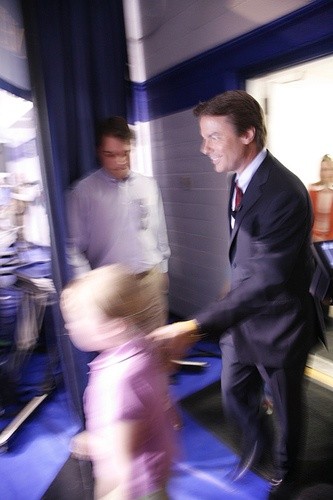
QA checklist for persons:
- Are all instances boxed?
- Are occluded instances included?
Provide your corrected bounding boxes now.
[60,120,169,348]
[58,261,180,500]
[139,88,328,500]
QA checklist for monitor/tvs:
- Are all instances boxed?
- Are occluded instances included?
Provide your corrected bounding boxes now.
[307,238,333,307]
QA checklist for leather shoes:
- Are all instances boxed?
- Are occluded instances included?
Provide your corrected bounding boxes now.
[266,461,302,500]
[228,434,274,487]
